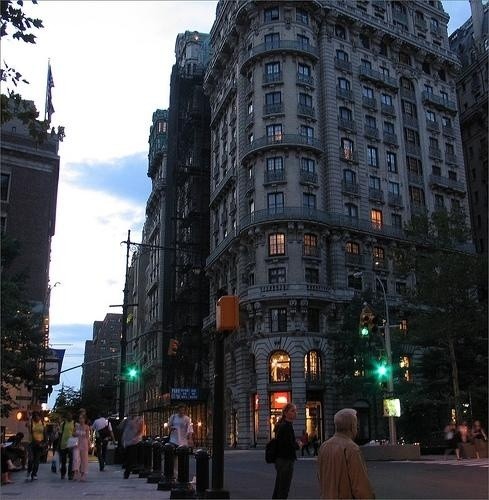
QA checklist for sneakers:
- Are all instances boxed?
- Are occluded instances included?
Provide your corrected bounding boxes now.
[52,463,87,481]
[2,466,38,486]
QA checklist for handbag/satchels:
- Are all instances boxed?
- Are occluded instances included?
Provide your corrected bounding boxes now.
[67,437,79,449]
[98,428,110,440]
[265,439,279,464]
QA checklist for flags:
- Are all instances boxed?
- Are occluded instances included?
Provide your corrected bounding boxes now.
[46,62,55,123]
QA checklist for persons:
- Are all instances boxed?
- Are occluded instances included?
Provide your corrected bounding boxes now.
[299,429,318,457]
[0,408,94,484]
[316,409,374,499]
[167,406,194,480]
[92,410,117,471]
[442,418,487,461]
[272,404,300,499]
[119,410,145,479]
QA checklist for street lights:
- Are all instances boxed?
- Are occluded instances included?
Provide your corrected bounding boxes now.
[353,270,398,445]
